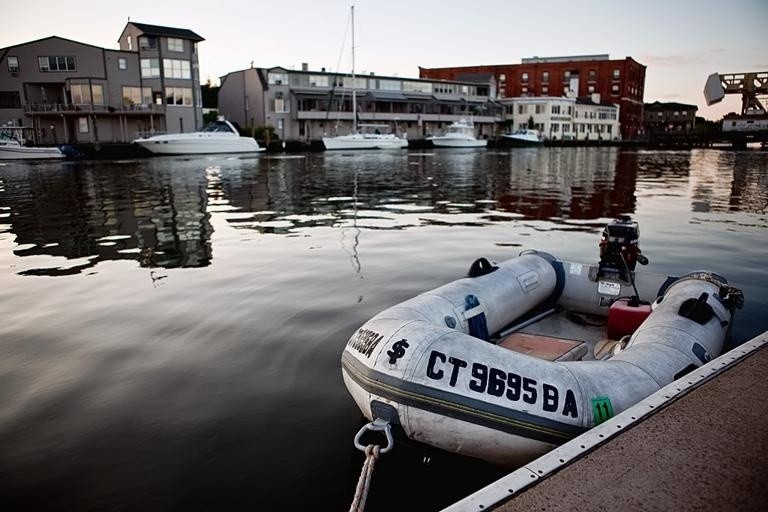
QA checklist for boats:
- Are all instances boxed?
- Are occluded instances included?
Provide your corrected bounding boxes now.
[130,116,267,155]
[425,115,488,148]
[0,121,67,160]
[342,249,745,466]
[496,129,541,146]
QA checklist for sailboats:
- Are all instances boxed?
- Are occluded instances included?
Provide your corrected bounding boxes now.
[321,6,409,150]
